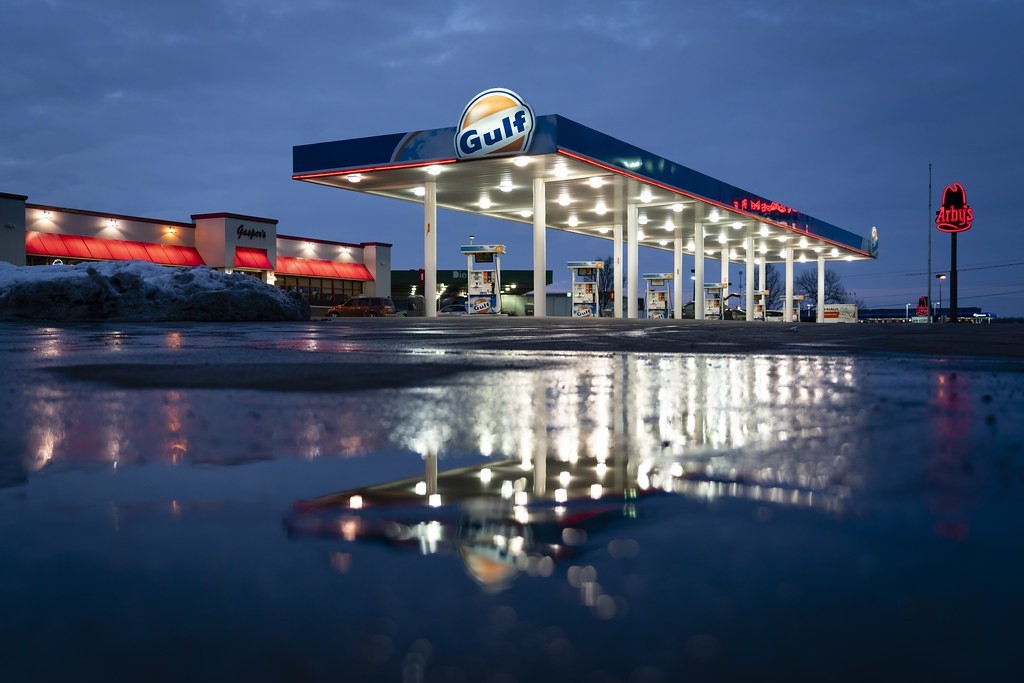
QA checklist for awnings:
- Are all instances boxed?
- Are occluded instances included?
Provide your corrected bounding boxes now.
[274,254,375,282]
[233,247,274,271]
[25,230,207,268]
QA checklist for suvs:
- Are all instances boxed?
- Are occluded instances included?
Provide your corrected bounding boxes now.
[326,297,396,318]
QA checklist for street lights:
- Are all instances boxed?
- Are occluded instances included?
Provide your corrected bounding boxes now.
[933,300,939,322]
[905,301,912,320]
[938,273,946,316]
[689,275,697,303]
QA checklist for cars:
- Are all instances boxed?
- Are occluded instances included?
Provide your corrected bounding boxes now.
[437,301,471,316]
